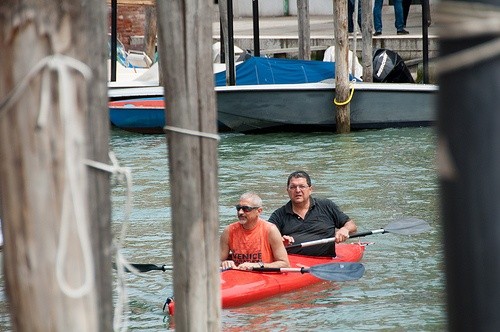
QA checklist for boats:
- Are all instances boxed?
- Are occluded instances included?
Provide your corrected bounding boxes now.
[168,242,375,315]
[108,84,438,134]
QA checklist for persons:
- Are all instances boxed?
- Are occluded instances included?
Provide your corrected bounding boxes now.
[268,171,357,257]
[348,0,362,35]
[218,193,290,271]
[372,0,409,36]
[402,0,431,27]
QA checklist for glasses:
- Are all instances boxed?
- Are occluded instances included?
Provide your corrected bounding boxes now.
[287,184,309,191]
[236,205,259,212]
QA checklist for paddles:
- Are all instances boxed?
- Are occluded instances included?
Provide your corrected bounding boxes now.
[111,261,365,282]
[284,216,430,251]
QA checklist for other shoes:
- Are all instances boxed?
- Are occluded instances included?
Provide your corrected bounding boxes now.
[397,29,410,35]
[372,31,382,36]
